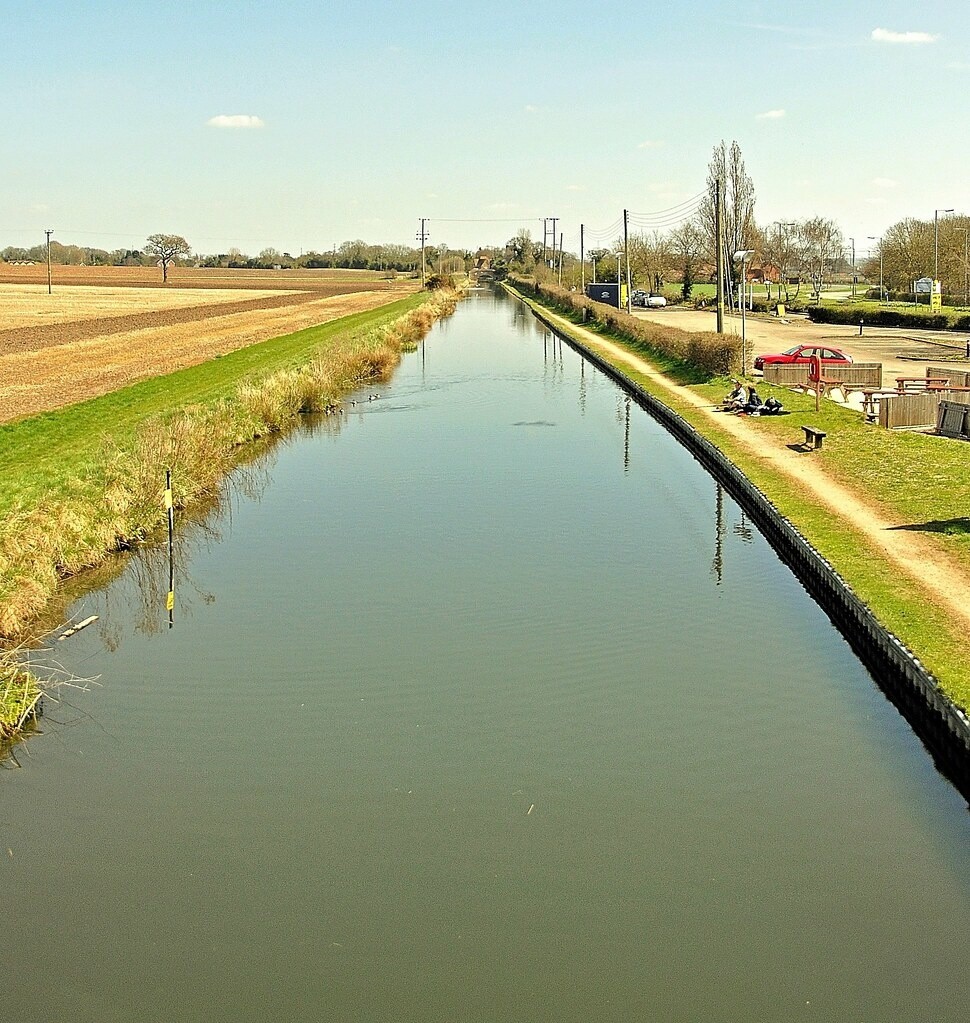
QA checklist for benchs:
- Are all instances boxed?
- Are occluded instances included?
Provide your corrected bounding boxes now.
[867,397,880,401]
[801,426,827,449]
[927,386,970,391]
[894,387,926,391]
[859,401,880,404]
[844,388,865,396]
[905,383,930,387]
[798,383,822,397]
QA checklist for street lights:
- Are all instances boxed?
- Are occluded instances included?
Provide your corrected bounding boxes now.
[779,222,796,301]
[848,237,855,301]
[866,236,884,302]
[934,208,956,280]
[614,252,626,310]
[952,226,968,307]
[590,254,598,284]
[733,248,755,379]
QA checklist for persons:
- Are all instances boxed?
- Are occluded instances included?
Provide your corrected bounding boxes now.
[724,381,783,415]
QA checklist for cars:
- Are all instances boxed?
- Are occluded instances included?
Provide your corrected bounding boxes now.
[626,289,667,308]
[753,342,853,370]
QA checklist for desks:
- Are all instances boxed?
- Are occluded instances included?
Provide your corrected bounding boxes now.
[862,389,922,413]
[807,375,849,403]
[895,377,950,391]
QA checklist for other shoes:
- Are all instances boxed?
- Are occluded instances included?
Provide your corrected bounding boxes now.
[725,407,735,412]
